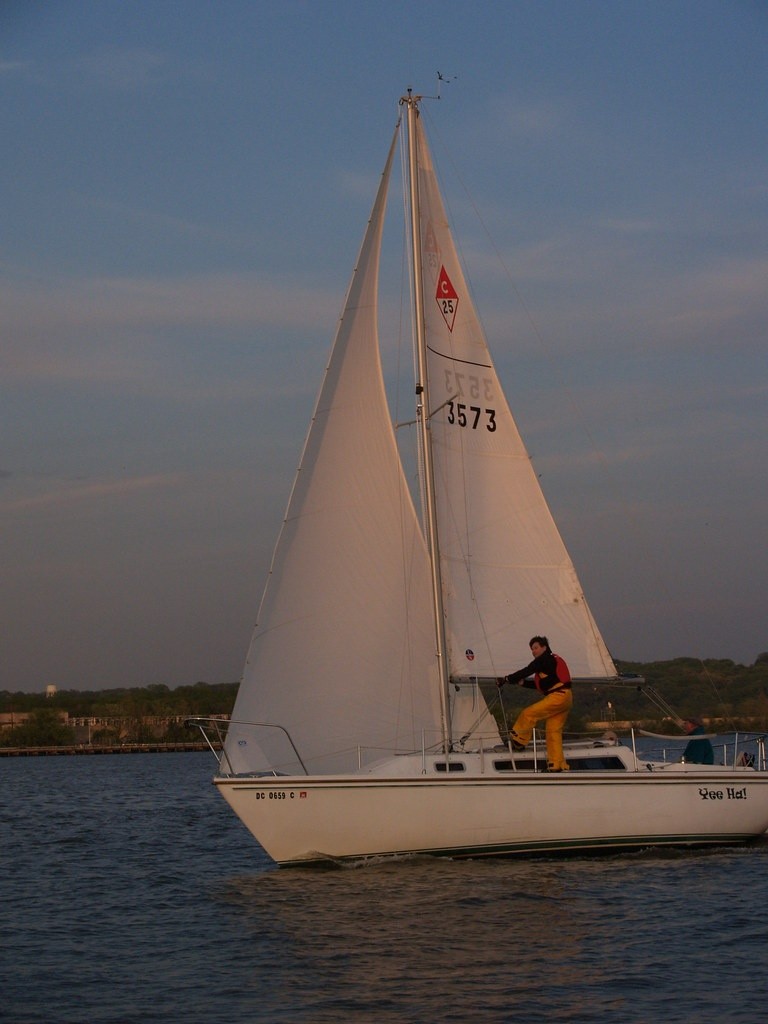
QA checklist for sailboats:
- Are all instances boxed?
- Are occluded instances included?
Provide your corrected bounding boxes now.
[182,90,768,867]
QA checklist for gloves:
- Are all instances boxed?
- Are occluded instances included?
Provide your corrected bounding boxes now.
[496,676,505,687]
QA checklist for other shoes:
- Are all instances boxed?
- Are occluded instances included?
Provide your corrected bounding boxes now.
[541,769,561,773]
[510,731,523,748]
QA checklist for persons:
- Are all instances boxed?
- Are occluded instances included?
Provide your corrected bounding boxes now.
[601,731,622,746]
[681,712,715,764]
[496,636,574,772]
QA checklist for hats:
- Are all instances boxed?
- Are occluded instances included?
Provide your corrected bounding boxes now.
[686,715,705,727]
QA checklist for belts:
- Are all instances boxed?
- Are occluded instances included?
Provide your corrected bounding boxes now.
[556,690,566,694]
